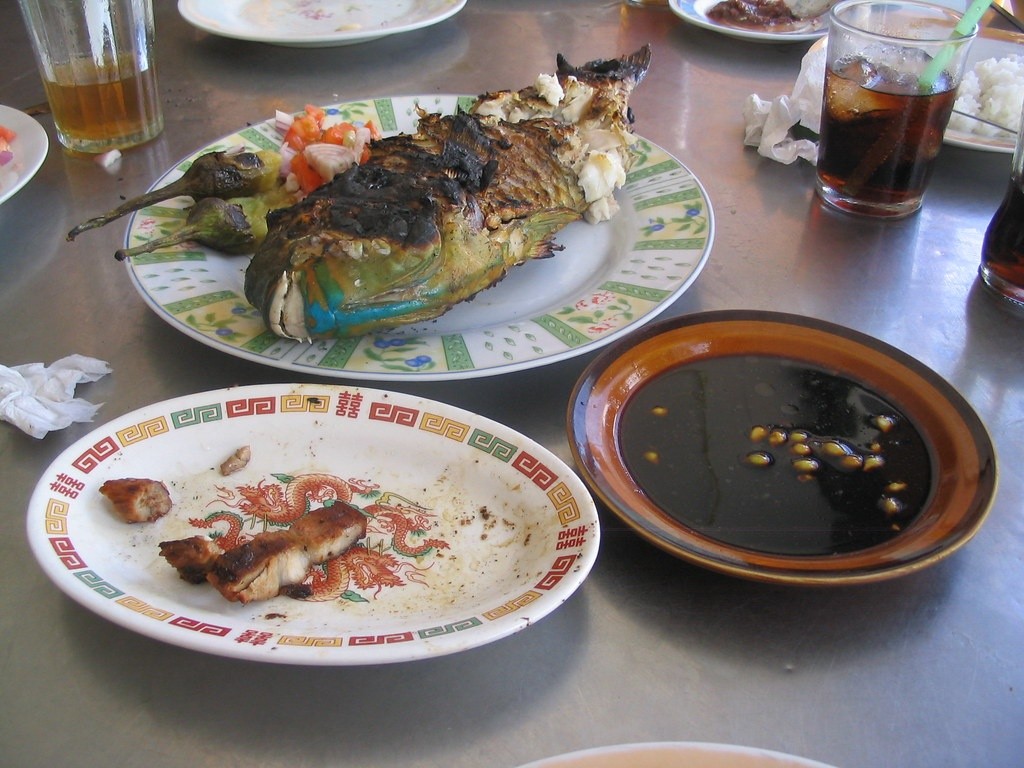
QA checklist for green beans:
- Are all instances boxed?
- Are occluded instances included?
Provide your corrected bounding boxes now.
[644,405,907,519]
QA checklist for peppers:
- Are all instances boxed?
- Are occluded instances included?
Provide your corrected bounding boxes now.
[66,149,283,241]
[113,183,299,262]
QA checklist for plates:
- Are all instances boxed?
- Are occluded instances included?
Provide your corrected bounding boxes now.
[121,95,717,382]
[27,384,600,666]
[511,741,838,768]
[177,0,467,48]
[0,104,50,206]
[566,308,999,588]
[668,0,832,43]
[806,36,1024,152]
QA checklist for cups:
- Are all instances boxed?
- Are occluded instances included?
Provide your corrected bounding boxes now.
[815,0,979,220]
[976,97,1024,311]
[18,0,166,153]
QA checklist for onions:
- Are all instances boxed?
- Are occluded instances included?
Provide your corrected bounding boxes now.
[274,109,371,182]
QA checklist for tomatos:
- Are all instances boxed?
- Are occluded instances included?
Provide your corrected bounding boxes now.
[283,103,380,194]
